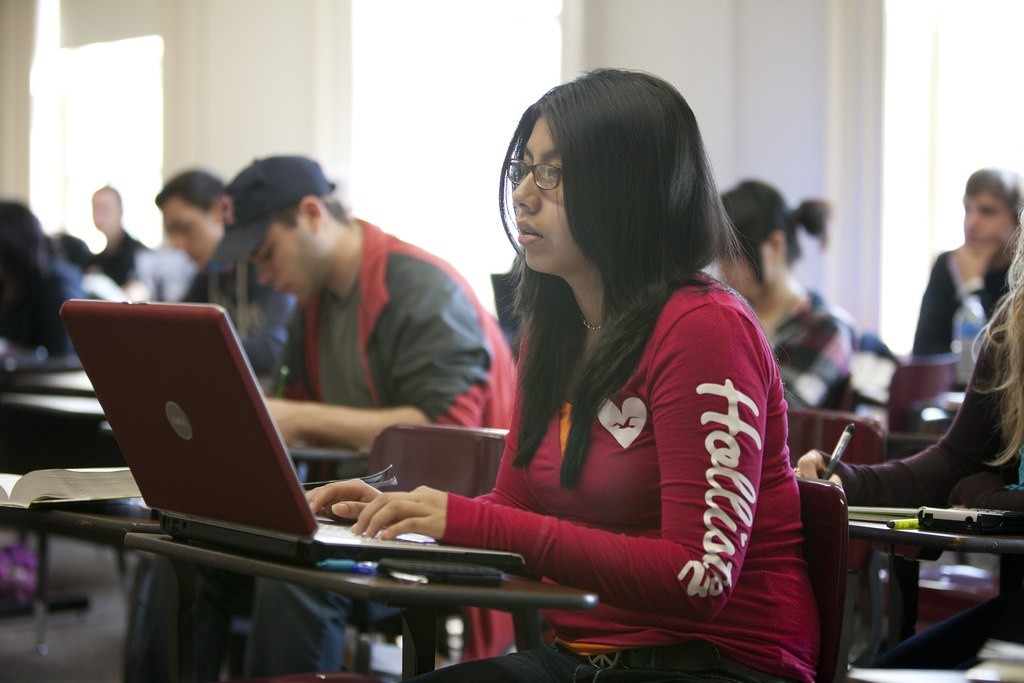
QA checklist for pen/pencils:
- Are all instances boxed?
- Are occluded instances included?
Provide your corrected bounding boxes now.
[821,423,855,480]
[887,519,920,529]
[275,366,291,397]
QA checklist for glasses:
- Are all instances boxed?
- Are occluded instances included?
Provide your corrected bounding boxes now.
[507,158,565,191]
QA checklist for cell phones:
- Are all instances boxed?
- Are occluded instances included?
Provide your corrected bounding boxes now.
[376,556,503,588]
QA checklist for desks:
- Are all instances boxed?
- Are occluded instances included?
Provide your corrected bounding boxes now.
[1,371,1023,683]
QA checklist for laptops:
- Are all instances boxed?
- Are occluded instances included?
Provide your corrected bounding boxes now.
[59,299,525,572]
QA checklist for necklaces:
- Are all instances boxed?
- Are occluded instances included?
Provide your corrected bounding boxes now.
[582,317,601,331]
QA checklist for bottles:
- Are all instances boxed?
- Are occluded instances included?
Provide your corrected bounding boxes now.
[951,293,983,383]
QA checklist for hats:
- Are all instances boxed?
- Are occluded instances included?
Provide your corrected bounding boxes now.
[205,156,334,261]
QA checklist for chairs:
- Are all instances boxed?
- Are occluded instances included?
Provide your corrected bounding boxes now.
[886,357,960,435]
[792,474,849,683]
[368,423,510,683]
[783,406,887,669]
[371,358,997,683]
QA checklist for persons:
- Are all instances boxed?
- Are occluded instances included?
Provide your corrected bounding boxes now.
[912,167,1024,391]
[126,155,518,683]
[155,170,297,375]
[0,203,90,355]
[305,68,820,683]
[83,185,152,285]
[798,210,1024,671]
[715,180,854,409]
